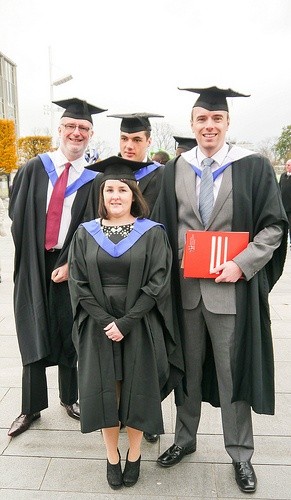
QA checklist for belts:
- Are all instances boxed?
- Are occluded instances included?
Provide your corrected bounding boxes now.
[48,248,62,253]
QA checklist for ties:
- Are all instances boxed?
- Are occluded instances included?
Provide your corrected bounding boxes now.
[44,162,71,251]
[197,158,215,228]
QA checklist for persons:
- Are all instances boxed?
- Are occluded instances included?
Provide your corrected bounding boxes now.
[81,112,165,443]
[152,151,171,165]
[278,159,291,248]
[150,87,289,493]
[67,155,177,491]
[7,97,109,436]
[173,136,198,156]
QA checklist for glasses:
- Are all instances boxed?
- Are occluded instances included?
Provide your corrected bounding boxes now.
[61,123,92,135]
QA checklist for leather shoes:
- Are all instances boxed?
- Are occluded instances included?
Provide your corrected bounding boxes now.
[60,402,81,419]
[232,461,257,492]
[157,442,196,467]
[142,432,159,442]
[8,411,40,437]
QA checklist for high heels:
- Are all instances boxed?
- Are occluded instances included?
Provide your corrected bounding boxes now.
[123,448,141,487]
[106,448,123,489]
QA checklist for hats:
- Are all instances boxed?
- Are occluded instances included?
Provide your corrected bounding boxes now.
[177,86,252,113]
[106,111,165,134]
[52,97,108,123]
[175,136,197,152]
[83,156,154,184]
